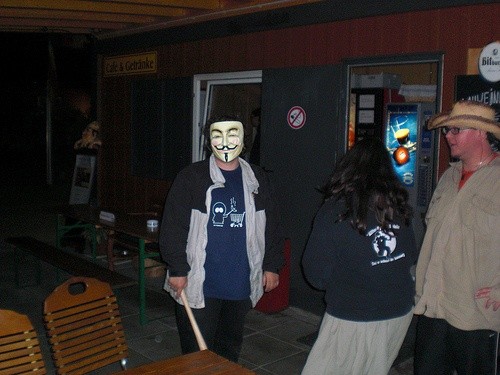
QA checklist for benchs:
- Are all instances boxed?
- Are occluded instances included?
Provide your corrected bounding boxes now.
[5,234,133,289]
[114,231,167,264]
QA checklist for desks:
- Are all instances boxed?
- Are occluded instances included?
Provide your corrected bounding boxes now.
[52,205,160,326]
[105,350,258,375]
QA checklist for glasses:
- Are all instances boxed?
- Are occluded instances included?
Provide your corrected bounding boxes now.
[441,126,477,136]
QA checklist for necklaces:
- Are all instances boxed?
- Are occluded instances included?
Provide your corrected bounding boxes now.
[463,150,495,174]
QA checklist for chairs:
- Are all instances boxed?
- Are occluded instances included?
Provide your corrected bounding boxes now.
[0,309,47,375]
[43,276,129,375]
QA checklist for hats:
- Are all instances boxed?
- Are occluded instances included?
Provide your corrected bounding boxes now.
[427,100,500,139]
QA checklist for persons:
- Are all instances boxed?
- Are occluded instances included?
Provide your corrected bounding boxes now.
[158,111,287,361]
[248,107,261,166]
[74,120,104,149]
[414,98,499,375]
[299,135,417,375]
